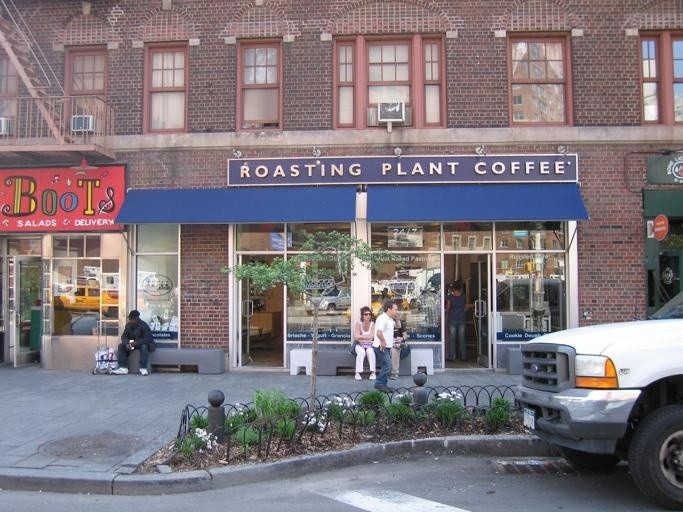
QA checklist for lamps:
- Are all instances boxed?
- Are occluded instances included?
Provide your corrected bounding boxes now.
[557,144,570,164]
[232,147,249,166]
[393,147,407,169]
[475,143,490,163]
[309,146,325,166]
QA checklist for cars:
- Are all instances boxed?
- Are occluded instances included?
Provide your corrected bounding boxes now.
[54,276,119,317]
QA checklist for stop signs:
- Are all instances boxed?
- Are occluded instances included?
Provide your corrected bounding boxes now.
[652,214,669,241]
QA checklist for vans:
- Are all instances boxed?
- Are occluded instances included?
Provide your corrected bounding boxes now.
[496,279,564,311]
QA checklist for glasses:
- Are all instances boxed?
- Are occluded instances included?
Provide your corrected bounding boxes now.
[363,312,372,316]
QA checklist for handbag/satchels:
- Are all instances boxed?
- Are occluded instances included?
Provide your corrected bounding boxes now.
[351,340,358,354]
[94,345,119,369]
[147,340,155,351]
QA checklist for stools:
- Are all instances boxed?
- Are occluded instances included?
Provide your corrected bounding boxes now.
[410,347,435,375]
[288,348,313,376]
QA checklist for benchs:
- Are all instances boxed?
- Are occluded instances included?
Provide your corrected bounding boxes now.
[128,347,225,376]
[506,348,523,374]
[313,348,391,377]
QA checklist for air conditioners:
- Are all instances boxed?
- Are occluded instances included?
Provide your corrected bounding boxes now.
[374,99,408,125]
[0,115,13,137]
[69,115,96,134]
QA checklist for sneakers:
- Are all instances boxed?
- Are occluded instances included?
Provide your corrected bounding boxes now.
[390,373,399,380]
[395,343,408,350]
[140,368,148,375]
[111,368,129,375]
[355,373,362,380]
[369,372,376,380]
[374,383,394,392]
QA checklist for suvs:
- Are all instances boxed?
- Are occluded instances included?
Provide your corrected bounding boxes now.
[305,286,350,316]
[389,281,418,309]
[513,289,683,512]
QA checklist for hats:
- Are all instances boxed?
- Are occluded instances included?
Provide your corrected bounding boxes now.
[128,311,140,317]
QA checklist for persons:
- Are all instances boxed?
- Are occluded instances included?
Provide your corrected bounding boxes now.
[444,279,468,362]
[384,314,404,380]
[109,309,156,376]
[368,301,398,394]
[349,306,377,381]
